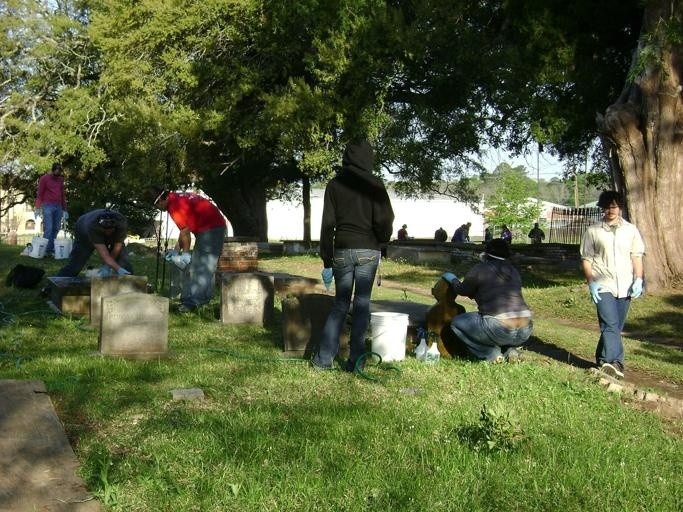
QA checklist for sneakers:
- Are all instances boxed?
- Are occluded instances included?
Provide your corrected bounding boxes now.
[177,302,198,313]
[601,359,624,379]
[487,349,520,364]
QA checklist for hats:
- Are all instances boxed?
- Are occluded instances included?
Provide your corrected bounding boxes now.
[142,186,165,206]
[484,237,510,261]
[52,163,64,172]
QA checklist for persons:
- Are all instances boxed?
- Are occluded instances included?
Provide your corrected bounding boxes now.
[441,239,535,364]
[579,191,645,380]
[310,138,395,373]
[450,225,468,243]
[435,227,448,243]
[39,208,135,300]
[500,225,513,245]
[144,185,227,313]
[465,222,472,243]
[528,223,545,245]
[484,228,494,242]
[34,163,68,260]
[398,224,410,242]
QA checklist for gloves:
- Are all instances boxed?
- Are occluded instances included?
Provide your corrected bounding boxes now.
[117,268,129,275]
[181,254,191,264]
[34,208,40,219]
[589,282,604,304]
[166,250,178,262]
[321,267,332,285]
[441,272,457,283]
[63,211,69,219]
[629,277,643,299]
[98,265,113,276]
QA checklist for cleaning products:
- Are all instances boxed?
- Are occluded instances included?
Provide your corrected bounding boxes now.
[415,328,430,361]
[425,330,441,362]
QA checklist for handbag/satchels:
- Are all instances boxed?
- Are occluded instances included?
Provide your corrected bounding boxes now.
[4,264,46,289]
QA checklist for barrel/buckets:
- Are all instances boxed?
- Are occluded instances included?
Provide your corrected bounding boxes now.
[369,312,409,362]
[28,237,49,258]
[53,239,72,259]
